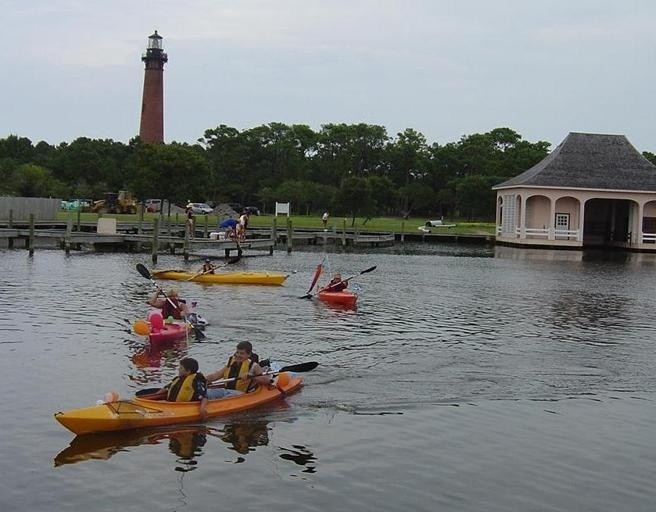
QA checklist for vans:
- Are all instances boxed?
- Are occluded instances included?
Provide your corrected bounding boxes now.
[142,198,161,213]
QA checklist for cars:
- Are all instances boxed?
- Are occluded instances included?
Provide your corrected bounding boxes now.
[230,203,246,212]
[207,200,219,208]
[184,202,216,215]
[61,198,96,210]
[245,206,264,215]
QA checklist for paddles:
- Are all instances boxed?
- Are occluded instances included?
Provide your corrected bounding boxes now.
[136,362,319,396]
[136,263,204,339]
[188,258,239,281]
[300,265,377,300]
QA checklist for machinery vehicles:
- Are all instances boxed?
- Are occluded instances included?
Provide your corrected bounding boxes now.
[80,191,142,215]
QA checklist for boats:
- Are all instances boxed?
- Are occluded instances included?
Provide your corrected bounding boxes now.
[316,283,359,306]
[146,312,195,344]
[54,373,305,434]
[150,267,292,287]
[132,343,187,367]
[50,403,300,467]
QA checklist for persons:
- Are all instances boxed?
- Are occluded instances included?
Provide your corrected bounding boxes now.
[185,206,249,241]
[322,209,330,228]
[149,285,189,323]
[324,272,348,292]
[196,259,226,275]
[204,341,271,399]
[155,357,209,423]
